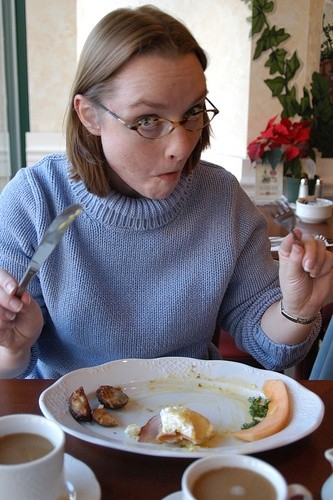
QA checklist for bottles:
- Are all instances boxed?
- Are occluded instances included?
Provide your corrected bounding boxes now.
[299,178,308,198]
[313,176,320,197]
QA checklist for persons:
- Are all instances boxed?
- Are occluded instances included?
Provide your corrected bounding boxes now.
[0,4,333,379]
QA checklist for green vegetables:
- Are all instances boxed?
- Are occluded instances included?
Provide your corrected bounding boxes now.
[240,396,270,429]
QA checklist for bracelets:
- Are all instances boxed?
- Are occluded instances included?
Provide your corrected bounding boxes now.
[280,299,322,324]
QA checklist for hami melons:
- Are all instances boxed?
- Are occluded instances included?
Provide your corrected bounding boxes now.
[234,380,289,441]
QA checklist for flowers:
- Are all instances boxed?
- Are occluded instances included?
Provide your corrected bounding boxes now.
[247,115,317,177]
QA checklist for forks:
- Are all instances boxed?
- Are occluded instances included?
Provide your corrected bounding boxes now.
[264,195,305,249]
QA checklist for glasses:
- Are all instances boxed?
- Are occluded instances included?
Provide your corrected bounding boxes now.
[91,94,219,140]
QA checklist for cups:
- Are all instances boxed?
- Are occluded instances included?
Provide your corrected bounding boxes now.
[182,454,313,500]
[39,357,325,458]
[0,414,66,500]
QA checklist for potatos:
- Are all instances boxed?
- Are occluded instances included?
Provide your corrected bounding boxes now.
[68,384,129,426]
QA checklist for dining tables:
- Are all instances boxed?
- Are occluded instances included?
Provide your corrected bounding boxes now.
[0,379,333,500]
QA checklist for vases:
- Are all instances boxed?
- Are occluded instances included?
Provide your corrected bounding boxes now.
[283,174,318,202]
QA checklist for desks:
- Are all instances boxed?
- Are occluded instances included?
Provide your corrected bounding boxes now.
[255,197,333,261]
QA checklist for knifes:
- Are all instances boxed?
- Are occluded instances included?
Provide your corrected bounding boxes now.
[14,203,83,299]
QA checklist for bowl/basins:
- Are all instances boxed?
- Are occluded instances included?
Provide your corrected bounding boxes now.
[295,198,333,224]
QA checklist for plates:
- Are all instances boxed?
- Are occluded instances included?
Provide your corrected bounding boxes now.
[64,453,101,500]
[321,473,333,500]
[162,490,183,500]
